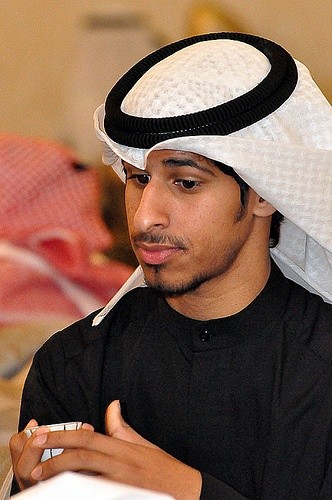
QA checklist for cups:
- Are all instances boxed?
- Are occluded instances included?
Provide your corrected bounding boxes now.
[24,421,82,462]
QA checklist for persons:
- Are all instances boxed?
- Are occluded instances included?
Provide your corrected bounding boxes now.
[0,30,332,500]
